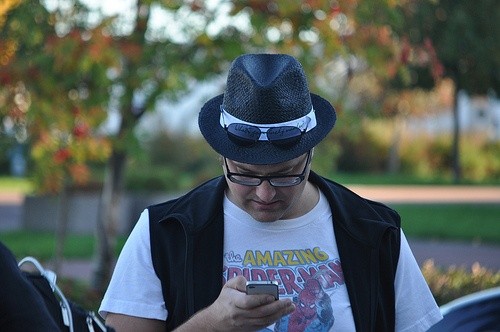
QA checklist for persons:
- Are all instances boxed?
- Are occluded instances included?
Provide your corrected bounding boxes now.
[93,50,445,332]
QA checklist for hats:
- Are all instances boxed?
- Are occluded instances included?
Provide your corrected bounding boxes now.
[198,53,337,165]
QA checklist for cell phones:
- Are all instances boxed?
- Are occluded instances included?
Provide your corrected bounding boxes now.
[245,280,279,302]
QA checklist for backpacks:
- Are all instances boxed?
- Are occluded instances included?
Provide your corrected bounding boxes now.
[16,255,117,332]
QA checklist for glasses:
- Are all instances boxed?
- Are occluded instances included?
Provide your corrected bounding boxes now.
[222,148,314,187]
